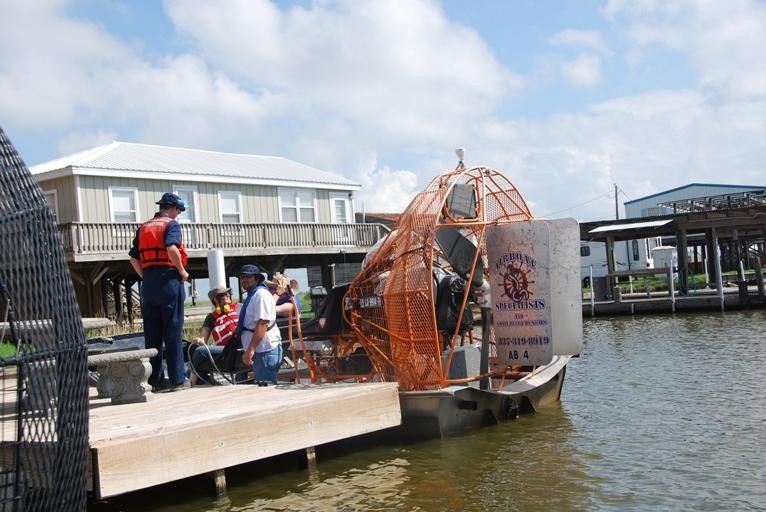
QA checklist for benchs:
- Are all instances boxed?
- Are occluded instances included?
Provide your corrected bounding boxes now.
[192,313,309,385]
[24,345,158,417]
[292,283,376,340]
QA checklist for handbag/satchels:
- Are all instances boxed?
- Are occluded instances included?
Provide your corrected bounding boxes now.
[216,334,244,374]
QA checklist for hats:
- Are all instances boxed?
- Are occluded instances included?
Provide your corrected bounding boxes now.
[264,272,301,295]
[208,286,232,302]
[234,264,265,284]
[154,192,187,212]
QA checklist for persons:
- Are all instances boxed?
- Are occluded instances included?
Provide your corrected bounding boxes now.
[127,192,193,394]
[233,264,285,381]
[189,271,302,385]
[193,286,248,384]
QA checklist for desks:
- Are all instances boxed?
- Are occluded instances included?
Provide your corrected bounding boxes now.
[0,317,114,359]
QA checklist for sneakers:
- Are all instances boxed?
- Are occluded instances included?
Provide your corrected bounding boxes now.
[170,376,191,392]
[151,378,169,392]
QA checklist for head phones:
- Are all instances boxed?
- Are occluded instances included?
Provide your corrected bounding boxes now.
[156,199,185,208]
[215,303,230,315]
[255,274,265,283]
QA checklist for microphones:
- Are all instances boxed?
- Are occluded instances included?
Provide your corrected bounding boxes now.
[241,279,259,295]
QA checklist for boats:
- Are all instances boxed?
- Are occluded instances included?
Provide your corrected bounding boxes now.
[276,148,584,440]
